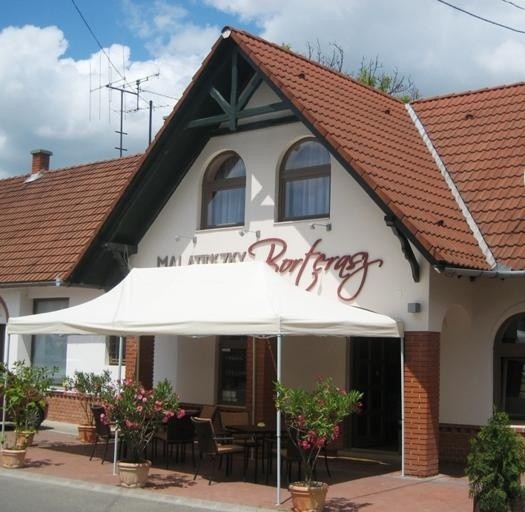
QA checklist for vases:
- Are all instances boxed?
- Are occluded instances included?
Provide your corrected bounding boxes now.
[117,460,151,488]
[288,481,328,512]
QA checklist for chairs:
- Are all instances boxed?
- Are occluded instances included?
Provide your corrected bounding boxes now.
[89,403,331,484]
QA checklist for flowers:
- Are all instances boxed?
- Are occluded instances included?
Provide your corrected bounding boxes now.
[271,377,363,485]
[99,379,185,462]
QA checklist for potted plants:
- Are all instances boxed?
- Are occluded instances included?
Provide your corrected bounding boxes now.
[0,360,45,469]
[64,370,111,442]
[13,367,59,447]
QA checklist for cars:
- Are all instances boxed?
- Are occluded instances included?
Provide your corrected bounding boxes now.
[0,365,48,429]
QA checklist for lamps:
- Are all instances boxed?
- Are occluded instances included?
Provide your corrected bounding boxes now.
[173,223,331,243]
